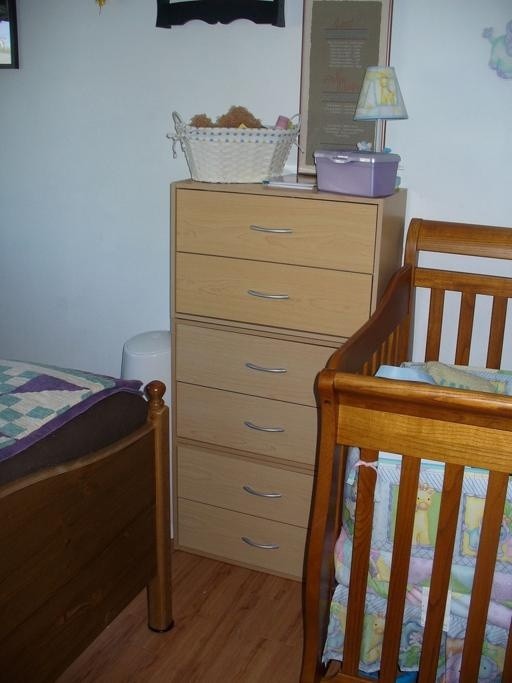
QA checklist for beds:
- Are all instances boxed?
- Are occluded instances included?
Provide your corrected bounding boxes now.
[1,354,174,682]
[302,216,512,682]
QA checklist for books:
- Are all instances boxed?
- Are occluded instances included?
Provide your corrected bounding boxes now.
[269,174,316,190]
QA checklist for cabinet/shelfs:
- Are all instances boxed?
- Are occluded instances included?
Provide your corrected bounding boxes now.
[170,171,407,585]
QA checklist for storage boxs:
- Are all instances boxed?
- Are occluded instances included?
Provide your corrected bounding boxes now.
[314,148,400,198]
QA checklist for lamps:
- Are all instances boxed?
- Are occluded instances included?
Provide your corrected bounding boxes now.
[352,64,409,188]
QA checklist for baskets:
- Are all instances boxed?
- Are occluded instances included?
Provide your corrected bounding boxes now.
[167,111,304,184]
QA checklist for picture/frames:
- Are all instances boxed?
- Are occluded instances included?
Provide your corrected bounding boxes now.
[297,1,393,176]
[0,0,18,69]
